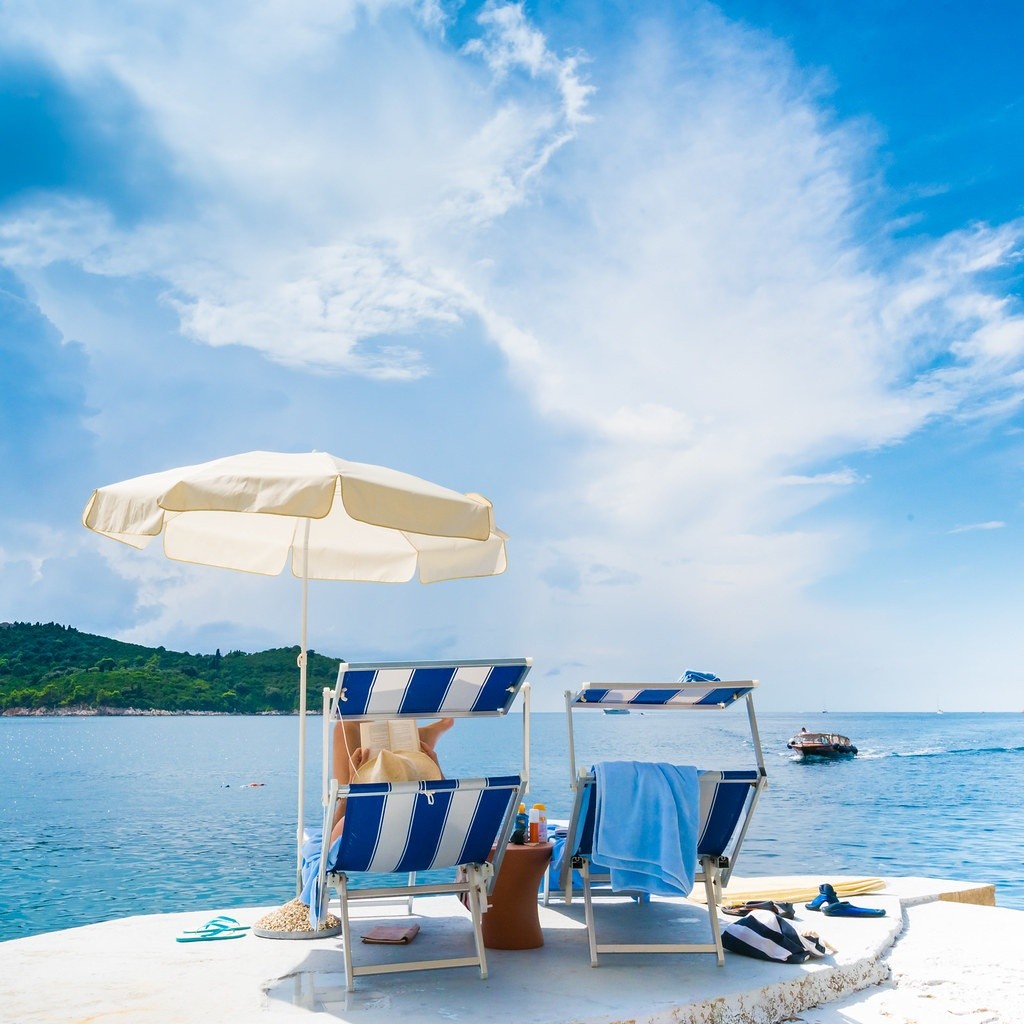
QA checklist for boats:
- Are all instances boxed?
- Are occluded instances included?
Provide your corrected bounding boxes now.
[787,727,859,756]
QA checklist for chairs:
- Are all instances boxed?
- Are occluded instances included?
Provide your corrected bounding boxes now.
[538,680,768,969]
[297,658,532,992]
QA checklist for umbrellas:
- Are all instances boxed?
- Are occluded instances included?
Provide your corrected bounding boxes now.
[81,451,512,897]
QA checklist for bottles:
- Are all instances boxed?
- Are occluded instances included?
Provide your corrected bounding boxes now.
[515,802,529,843]
[532,803,548,843]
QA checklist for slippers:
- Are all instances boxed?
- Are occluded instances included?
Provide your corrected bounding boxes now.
[184,915,251,933]
[176,917,245,941]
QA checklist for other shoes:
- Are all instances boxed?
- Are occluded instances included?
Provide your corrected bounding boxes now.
[721,900,795,920]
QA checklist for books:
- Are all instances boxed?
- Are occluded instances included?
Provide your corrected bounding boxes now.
[359,719,421,763]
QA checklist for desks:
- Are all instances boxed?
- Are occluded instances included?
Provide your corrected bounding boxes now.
[482,841,553,949]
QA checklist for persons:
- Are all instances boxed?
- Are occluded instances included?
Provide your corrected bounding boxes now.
[800,728,811,741]
[330,718,454,851]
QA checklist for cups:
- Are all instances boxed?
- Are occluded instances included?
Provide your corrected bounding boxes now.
[528,809,539,843]
